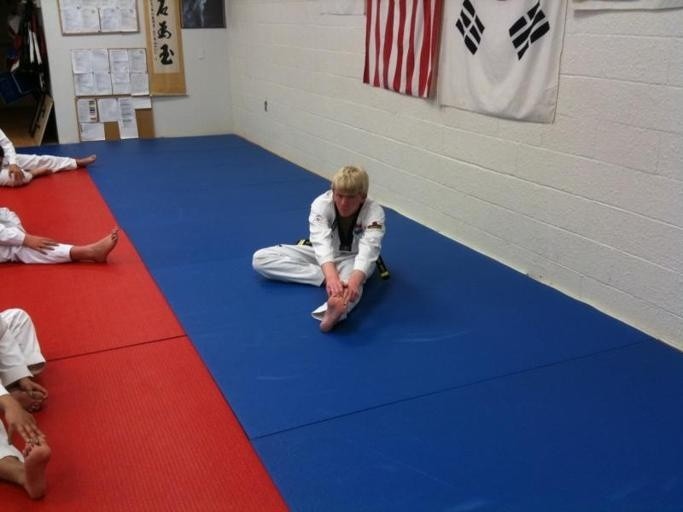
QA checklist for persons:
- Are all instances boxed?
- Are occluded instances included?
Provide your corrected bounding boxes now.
[250,166,387,334]
[0,128,99,187]
[0,306,49,412]
[0,378,51,500]
[0,205,118,265]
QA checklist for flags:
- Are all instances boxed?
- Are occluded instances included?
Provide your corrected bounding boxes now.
[437,0,568,123]
[363,0,438,100]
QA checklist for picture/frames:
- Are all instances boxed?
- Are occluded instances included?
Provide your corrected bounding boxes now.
[179,0,227,29]
